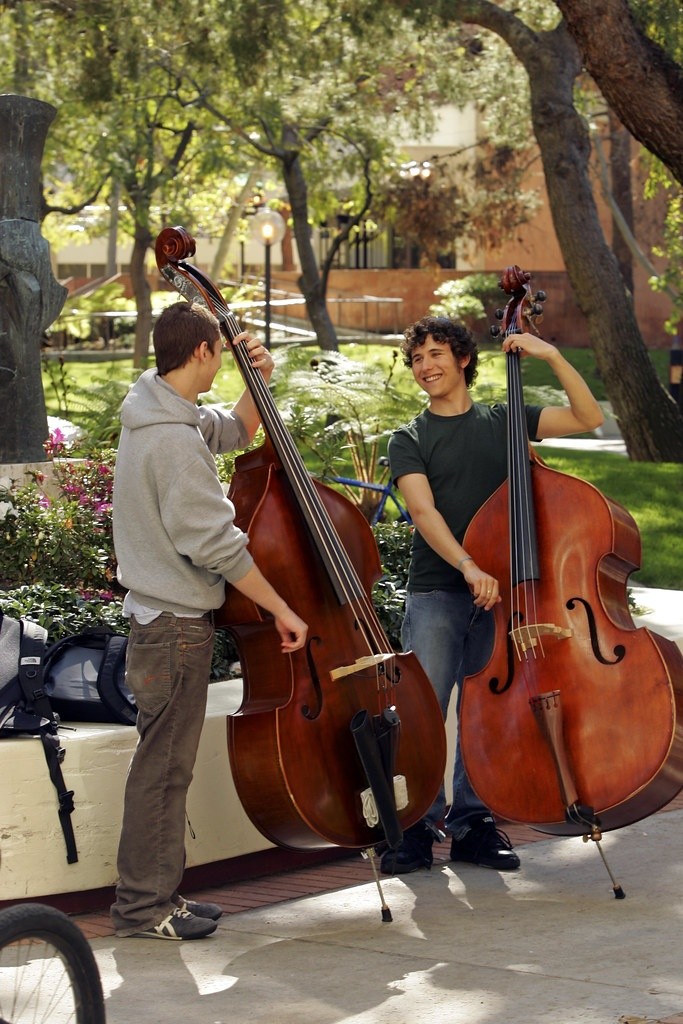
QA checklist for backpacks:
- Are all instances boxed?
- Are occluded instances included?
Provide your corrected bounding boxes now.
[0,606,139,739]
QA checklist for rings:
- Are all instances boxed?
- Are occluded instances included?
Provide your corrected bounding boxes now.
[487,591,492,594]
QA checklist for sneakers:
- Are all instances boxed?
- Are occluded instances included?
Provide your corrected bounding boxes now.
[129,896,223,940]
[450,813,520,870]
[379,819,434,875]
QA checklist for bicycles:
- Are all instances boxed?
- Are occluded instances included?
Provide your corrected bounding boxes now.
[0,903,107,1024]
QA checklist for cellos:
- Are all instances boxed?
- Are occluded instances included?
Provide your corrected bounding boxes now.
[145,219,445,920]
[452,263,683,911]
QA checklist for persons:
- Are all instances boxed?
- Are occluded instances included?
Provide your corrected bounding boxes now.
[109,302,308,941]
[379,316,606,876]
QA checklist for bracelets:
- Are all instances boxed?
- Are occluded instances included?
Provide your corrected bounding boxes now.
[458,558,472,570]
